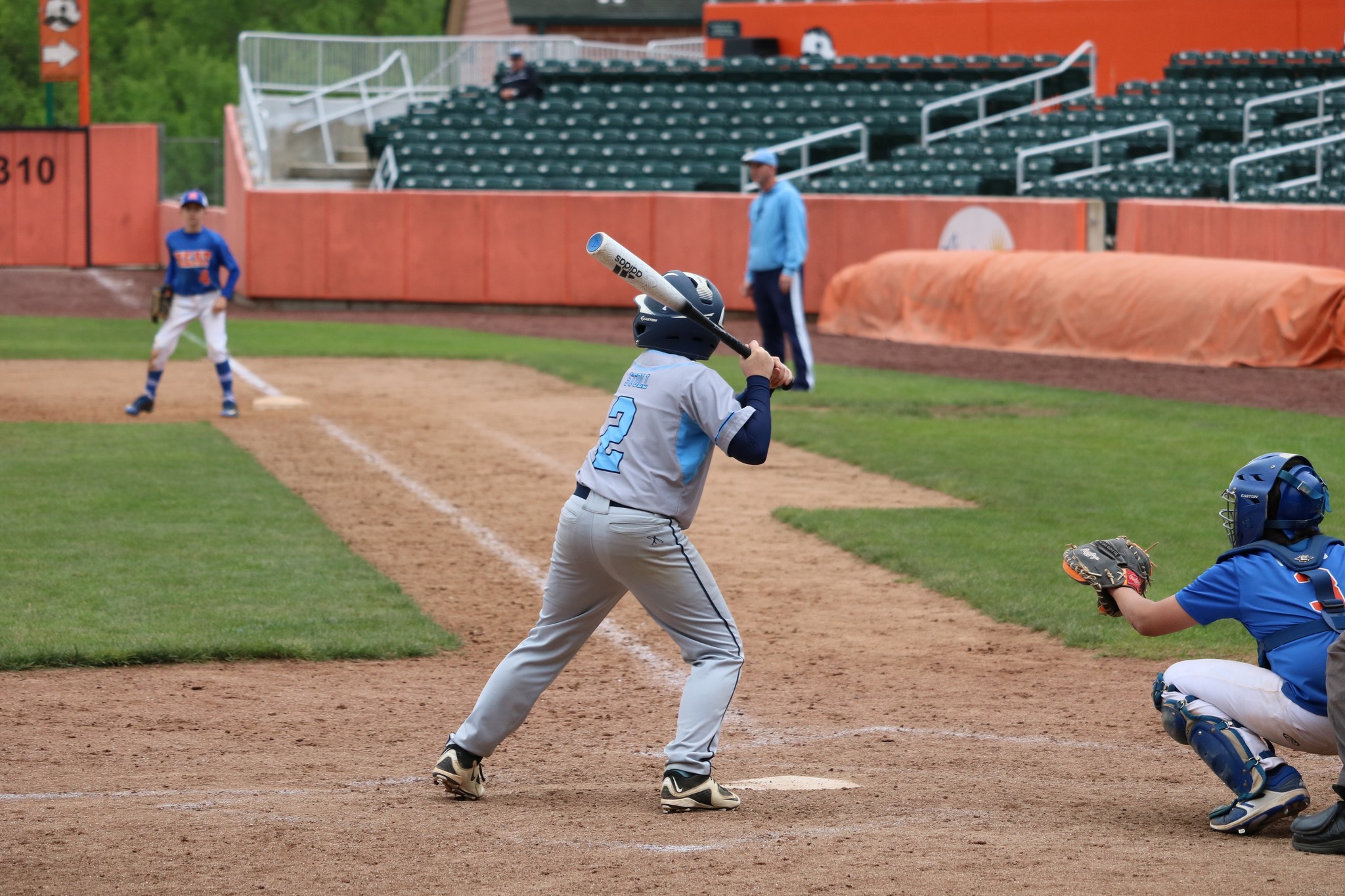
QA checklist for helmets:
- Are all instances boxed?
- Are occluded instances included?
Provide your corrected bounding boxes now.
[1219,452,1330,548]
[633,269,725,361]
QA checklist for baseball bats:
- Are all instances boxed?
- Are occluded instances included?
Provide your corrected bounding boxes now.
[588,231,793,393]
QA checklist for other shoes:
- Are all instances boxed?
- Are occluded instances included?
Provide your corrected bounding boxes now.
[125,395,152,414]
[222,400,238,417]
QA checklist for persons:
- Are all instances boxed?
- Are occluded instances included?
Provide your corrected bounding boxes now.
[432,267,793,815]
[740,147,813,391]
[498,53,545,102]
[124,190,242,420]
[1063,451,1345,855]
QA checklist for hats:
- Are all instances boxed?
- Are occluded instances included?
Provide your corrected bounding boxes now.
[742,149,777,168]
[181,192,208,208]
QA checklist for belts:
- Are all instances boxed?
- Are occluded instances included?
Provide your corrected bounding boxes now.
[574,483,630,509]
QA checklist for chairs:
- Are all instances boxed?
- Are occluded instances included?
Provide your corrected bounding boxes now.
[362,40,1345,201]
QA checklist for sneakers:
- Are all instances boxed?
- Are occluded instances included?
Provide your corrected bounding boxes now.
[1209,765,1311,834]
[1292,810,1345,852]
[431,744,485,800]
[660,769,741,814]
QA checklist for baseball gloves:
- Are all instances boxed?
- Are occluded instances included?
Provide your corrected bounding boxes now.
[1062,535,1153,618]
[147,285,173,324]
